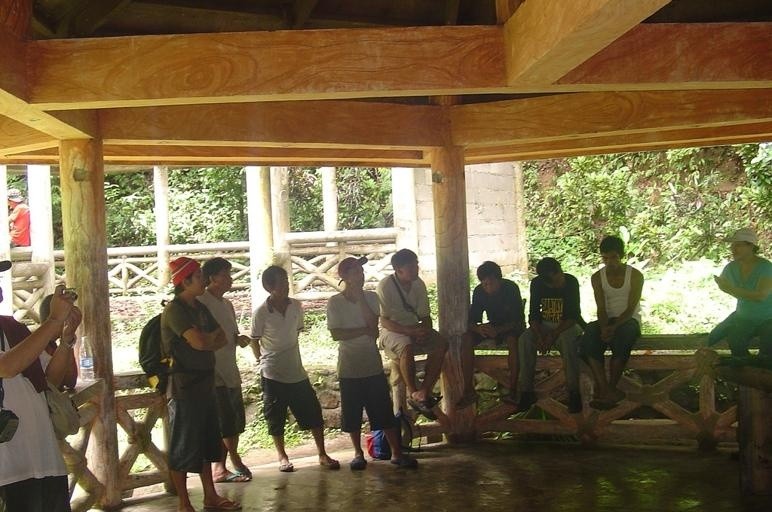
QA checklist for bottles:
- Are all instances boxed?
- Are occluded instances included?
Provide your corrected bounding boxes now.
[78,335,95,381]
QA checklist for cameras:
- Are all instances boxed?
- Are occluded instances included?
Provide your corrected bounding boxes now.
[63,289,78,300]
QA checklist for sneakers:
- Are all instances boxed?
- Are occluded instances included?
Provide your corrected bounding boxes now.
[566,395,582,414]
[515,391,539,411]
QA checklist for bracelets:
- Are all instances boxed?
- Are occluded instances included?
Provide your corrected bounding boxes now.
[61,335,77,350]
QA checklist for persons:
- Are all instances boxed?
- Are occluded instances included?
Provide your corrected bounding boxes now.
[40,296,77,390]
[158,256,242,512]
[377,248,449,422]
[580,235,644,409]
[714,229,772,363]
[455,262,526,411]
[326,255,418,469]
[252,265,340,471]
[197,256,253,482]
[515,257,580,414]
[0,262,81,511]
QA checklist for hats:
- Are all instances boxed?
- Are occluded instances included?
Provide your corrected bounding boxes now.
[0,260,12,273]
[7,188,25,203]
[338,256,368,278]
[170,257,201,287]
[722,227,759,247]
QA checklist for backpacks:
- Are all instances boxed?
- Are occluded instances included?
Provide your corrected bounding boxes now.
[365,411,402,459]
[139,314,181,394]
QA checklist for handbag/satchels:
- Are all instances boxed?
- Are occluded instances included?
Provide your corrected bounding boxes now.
[42,377,82,441]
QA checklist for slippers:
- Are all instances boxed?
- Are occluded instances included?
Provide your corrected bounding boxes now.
[454,393,478,409]
[590,389,627,411]
[279,459,293,472]
[350,457,366,470]
[390,456,418,469]
[408,391,443,420]
[319,456,340,466]
[203,497,243,510]
[212,465,252,482]
